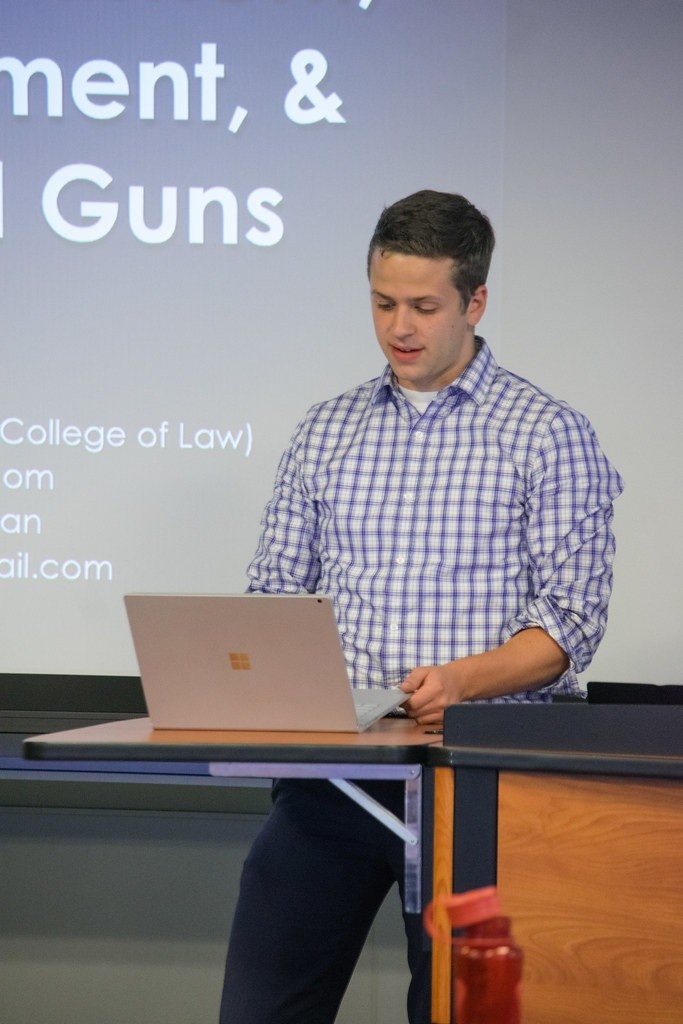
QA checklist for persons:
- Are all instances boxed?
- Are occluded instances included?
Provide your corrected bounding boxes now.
[220,188,625,1024]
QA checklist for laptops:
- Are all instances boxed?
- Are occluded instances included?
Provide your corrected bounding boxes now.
[123,594,414,733]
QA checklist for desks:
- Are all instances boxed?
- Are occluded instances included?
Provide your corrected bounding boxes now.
[0,714,451,1024]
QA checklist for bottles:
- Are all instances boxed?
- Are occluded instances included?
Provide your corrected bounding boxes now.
[423,886,526,1024]
